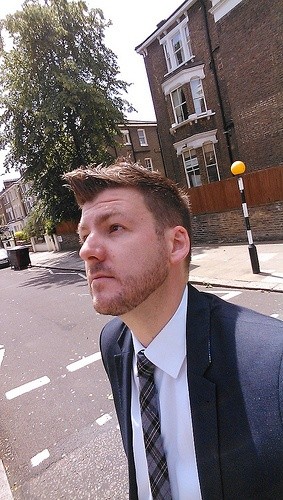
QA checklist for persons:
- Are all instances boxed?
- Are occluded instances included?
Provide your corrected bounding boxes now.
[63,164,283,500]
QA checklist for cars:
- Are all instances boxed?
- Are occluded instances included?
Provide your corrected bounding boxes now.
[0,247,10,268]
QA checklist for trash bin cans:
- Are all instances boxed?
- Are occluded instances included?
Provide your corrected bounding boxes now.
[7,246,31,271]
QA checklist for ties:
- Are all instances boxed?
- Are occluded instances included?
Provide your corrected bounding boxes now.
[137,349,173,500]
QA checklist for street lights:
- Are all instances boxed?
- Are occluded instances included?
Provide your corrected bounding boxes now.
[231,161,261,275]
